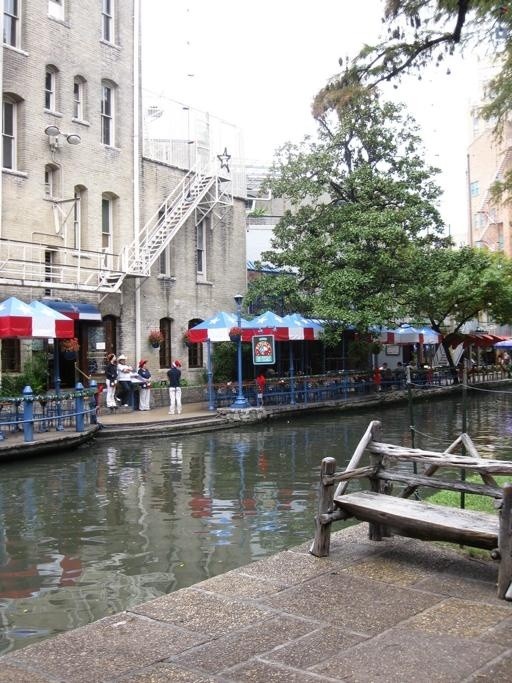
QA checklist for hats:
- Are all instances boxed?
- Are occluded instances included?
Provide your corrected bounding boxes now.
[172,360,181,368]
[116,354,129,361]
[138,359,147,367]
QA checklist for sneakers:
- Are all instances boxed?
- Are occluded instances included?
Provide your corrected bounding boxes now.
[168,411,174,414]
[110,404,129,409]
[140,408,149,411]
[177,410,181,414]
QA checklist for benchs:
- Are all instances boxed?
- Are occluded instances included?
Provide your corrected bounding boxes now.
[309,417,512,601]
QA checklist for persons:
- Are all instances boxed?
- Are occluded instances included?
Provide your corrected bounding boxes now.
[378,360,432,391]
[502,351,511,365]
[117,354,134,406]
[166,360,183,415]
[265,367,276,379]
[105,353,119,408]
[254,369,265,407]
[137,358,152,410]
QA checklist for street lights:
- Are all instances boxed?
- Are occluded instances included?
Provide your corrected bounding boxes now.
[233,292,244,404]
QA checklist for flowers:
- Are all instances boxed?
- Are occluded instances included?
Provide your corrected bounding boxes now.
[179,329,194,342]
[147,328,165,345]
[59,336,80,353]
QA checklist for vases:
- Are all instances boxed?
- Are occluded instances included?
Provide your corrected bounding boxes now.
[63,351,76,359]
[185,342,192,347]
[152,343,159,347]
